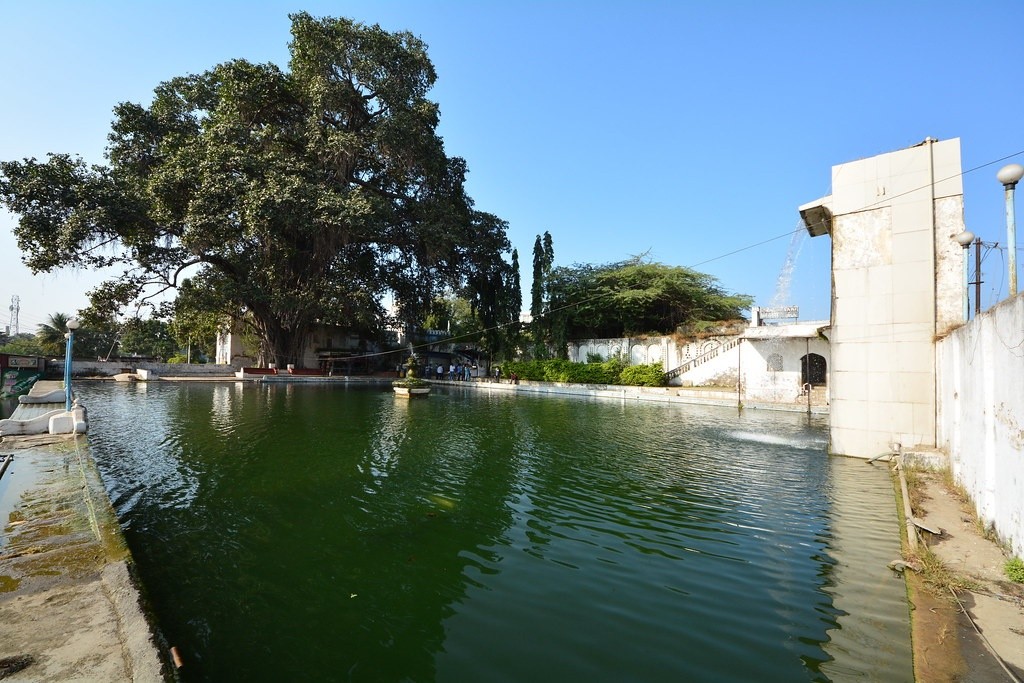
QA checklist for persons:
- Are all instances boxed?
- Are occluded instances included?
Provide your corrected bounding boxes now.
[416,362,422,377]
[510,372,518,385]
[396,362,402,377]
[425,363,471,381]
[496,366,500,383]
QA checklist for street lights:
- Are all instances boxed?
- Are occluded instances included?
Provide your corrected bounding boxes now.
[66,320,80,413]
[996,162,1024,296]
[959,231,975,326]
[64,333,70,389]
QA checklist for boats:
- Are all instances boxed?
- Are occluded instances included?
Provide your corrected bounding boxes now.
[393,386,431,397]
